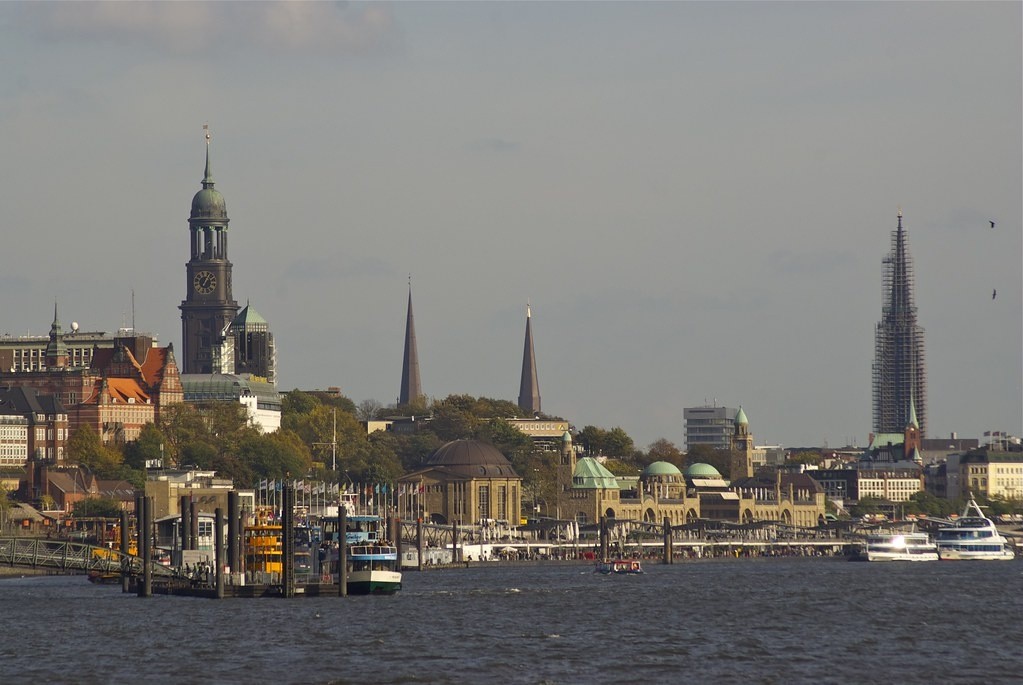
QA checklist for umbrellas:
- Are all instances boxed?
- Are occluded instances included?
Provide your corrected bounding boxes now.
[501,547,517,561]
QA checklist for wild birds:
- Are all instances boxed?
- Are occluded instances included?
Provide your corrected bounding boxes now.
[989,221,995,229]
[992,289,997,300]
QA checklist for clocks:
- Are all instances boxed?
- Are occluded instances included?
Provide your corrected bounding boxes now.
[193,271,217,295]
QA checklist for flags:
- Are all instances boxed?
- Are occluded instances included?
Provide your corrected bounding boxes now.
[254,479,426,496]
[984,431,1007,436]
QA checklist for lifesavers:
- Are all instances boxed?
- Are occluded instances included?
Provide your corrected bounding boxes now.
[322,574,330,582]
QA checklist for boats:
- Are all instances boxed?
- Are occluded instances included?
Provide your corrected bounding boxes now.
[309,493,403,595]
[242,507,310,578]
[937,491,1015,561]
[849,532,940,562]
[593,558,642,574]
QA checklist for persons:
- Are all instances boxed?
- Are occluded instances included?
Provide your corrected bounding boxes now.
[740,546,819,557]
[477,550,571,561]
[321,539,396,554]
[46,528,54,538]
[468,555,472,561]
[185,562,210,577]
